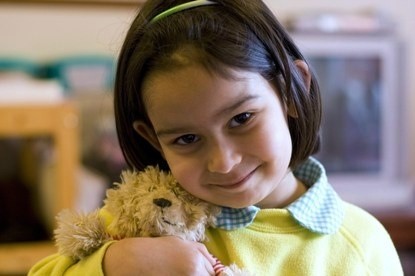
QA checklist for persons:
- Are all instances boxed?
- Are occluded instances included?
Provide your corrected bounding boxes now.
[26,0,404,276]
